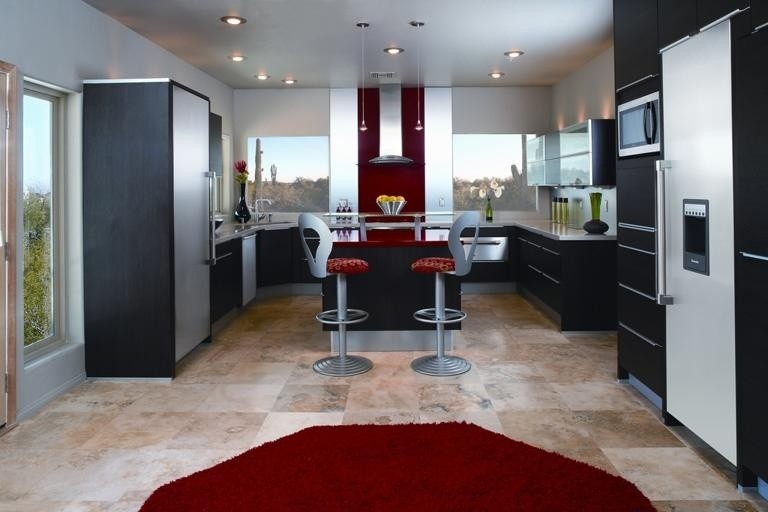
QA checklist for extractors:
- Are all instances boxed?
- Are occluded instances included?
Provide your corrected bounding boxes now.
[353,87,426,167]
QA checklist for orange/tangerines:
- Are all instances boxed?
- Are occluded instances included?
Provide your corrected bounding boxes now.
[377,194,405,202]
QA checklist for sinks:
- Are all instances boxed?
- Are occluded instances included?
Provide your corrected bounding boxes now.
[235,221,294,225]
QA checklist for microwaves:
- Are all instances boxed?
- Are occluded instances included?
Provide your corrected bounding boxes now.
[616,90,663,159]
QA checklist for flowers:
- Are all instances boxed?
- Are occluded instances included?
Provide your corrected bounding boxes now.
[468,178,506,199]
[234,161,249,184]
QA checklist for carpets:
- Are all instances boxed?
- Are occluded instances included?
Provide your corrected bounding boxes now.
[138,420,657,511]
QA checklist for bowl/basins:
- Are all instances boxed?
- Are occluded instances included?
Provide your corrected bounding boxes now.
[377,200,408,216]
[209,218,224,233]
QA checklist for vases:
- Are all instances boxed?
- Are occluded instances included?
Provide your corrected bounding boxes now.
[485,198,493,222]
[233,184,251,223]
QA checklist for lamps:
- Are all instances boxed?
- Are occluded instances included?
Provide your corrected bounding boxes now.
[357,21,426,132]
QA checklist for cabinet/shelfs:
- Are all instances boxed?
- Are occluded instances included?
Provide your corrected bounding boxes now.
[82,77,213,382]
[211,239,241,336]
[515,227,616,332]
[524,120,616,186]
[255,228,322,297]
[457,227,515,294]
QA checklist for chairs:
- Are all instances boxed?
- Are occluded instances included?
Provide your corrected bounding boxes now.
[297,211,481,375]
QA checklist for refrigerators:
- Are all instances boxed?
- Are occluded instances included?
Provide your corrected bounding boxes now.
[654,6,753,474]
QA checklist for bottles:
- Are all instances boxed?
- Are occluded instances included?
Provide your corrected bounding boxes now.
[551,196,570,225]
[331,229,350,242]
[485,198,493,224]
[335,206,352,220]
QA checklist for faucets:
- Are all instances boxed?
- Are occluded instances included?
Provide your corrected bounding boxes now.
[254,199,271,222]
